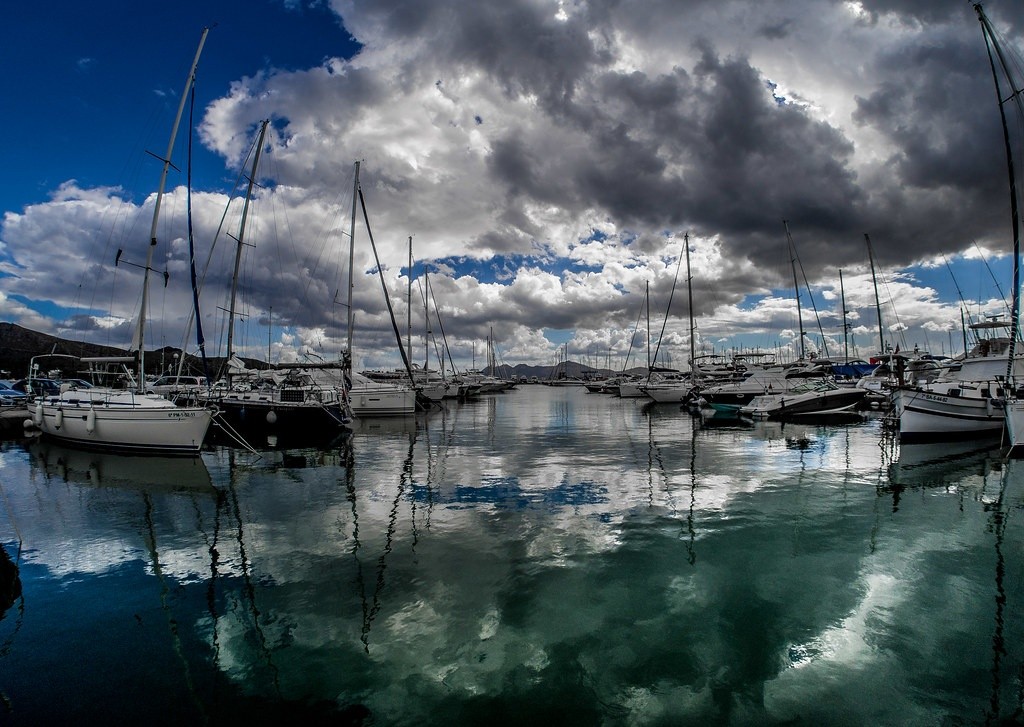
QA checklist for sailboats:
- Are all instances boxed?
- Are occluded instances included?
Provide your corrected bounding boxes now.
[0,26,516,457]
[545,1,1024,450]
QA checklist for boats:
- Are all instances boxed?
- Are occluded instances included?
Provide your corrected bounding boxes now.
[519,375,527,382]
[531,376,538,382]
